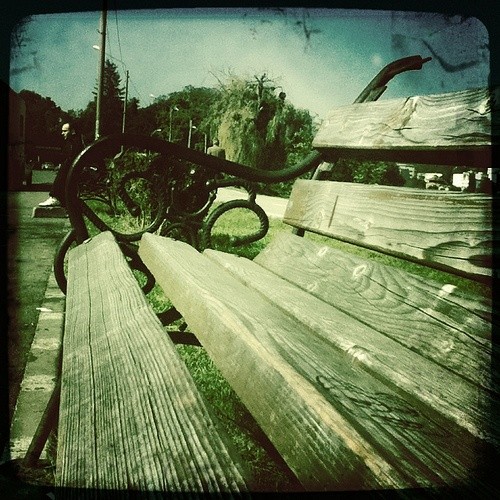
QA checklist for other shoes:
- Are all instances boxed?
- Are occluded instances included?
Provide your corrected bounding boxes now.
[39,196,61,206]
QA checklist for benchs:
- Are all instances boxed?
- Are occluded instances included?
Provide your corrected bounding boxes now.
[53,56,493,499]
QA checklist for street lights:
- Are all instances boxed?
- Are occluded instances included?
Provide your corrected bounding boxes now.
[174,106,192,148]
[148,93,173,142]
[91,44,130,134]
[192,125,207,153]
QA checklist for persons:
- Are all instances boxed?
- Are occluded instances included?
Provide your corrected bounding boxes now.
[38,120,106,210]
